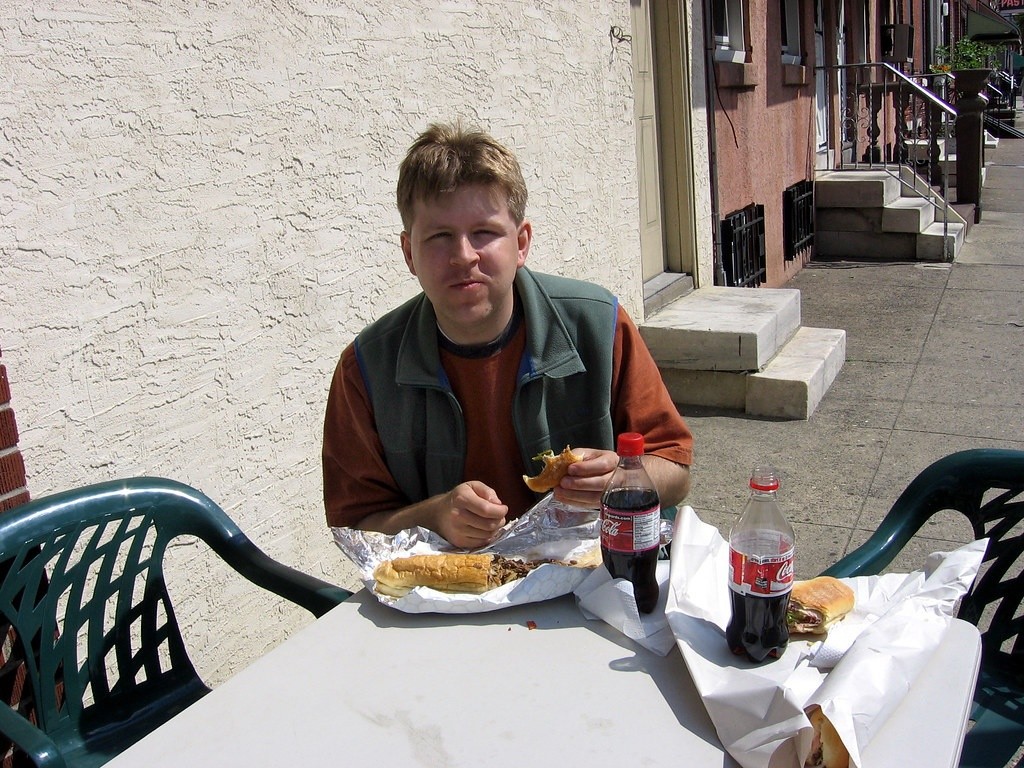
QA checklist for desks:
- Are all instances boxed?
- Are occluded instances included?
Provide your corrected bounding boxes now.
[100,578,982,768]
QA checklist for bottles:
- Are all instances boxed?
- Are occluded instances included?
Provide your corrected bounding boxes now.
[724,466,796,666]
[600,430,662,615]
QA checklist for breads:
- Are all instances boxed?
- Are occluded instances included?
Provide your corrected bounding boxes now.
[805,707,850,768]
[522,444,584,493]
[372,553,491,597]
[786,575,855,634]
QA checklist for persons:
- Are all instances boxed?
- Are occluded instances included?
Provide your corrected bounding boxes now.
[321,118,692,549]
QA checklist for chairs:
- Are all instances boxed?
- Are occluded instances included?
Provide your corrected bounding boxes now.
[822,446,1024,768]
[0,474,355,767]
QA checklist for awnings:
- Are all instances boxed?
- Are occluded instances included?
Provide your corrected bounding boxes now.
[967,2,1021,46]
